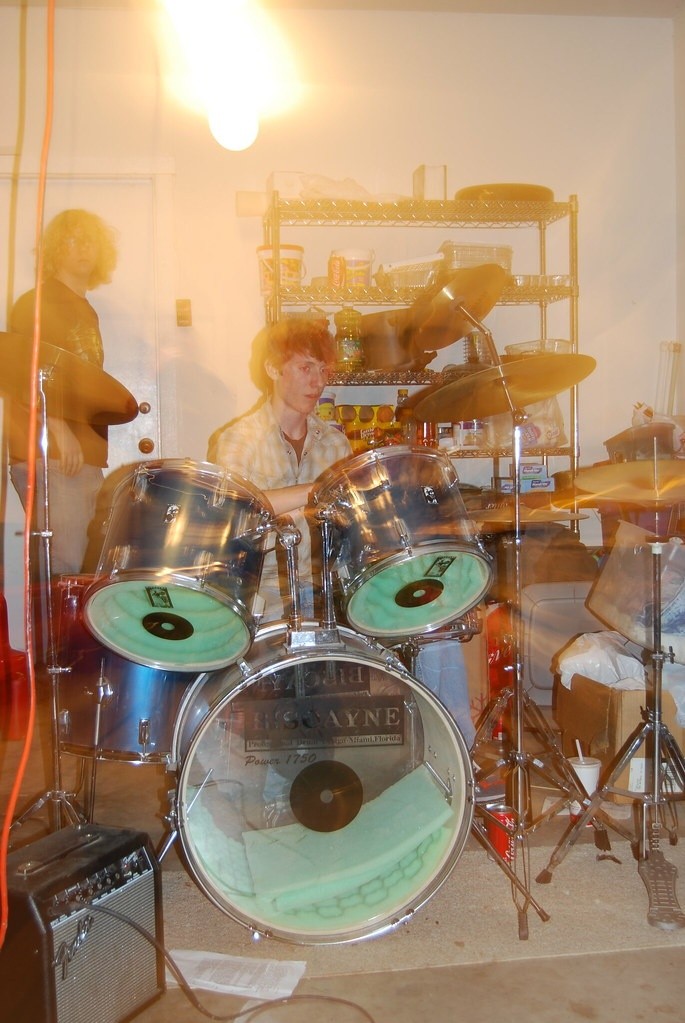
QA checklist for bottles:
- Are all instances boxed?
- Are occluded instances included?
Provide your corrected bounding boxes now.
[395,389,418,444]
[333,301,366,371]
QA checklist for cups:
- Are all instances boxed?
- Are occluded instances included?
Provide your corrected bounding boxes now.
[567,757,602,828]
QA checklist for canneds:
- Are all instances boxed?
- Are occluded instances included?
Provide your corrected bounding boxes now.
[328,255,346,289]
[487,805,518,862]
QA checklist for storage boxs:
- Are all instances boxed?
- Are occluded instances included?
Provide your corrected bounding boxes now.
[491,463,554,492]
[556,674,685,804]
[505,340,570,354]
[515,275,569,286]
[587,518,685,666]
[381,241,512,289]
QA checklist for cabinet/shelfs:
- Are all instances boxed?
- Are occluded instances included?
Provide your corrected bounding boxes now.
[263,191,580,540]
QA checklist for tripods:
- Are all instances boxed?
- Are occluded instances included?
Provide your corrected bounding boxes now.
[9,368,88,835]
[454,302,685,883]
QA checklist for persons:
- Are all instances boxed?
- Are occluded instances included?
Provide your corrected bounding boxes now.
[208,316,359,649]
[5,208,121,660]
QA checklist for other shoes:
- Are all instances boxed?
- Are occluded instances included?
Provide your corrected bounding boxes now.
[471,775,506,803]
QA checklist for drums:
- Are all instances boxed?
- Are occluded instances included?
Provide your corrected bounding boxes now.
[310,444,495,638]
[48,573,196,765]
[79,458,272,673]
[170,618,475,946]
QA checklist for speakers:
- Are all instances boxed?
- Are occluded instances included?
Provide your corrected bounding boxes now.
[0,822,167,1023]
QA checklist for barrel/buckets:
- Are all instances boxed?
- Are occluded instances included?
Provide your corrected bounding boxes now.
[313,392,335,423]
[257,245,307,295]
[331,247,375,285]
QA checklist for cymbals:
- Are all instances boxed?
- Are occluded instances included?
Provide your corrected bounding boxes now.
[413,354,596,423]
[470,504,591,522]
[573,459,685,509]
[0,331,139,425]
[398,264,507,352]
